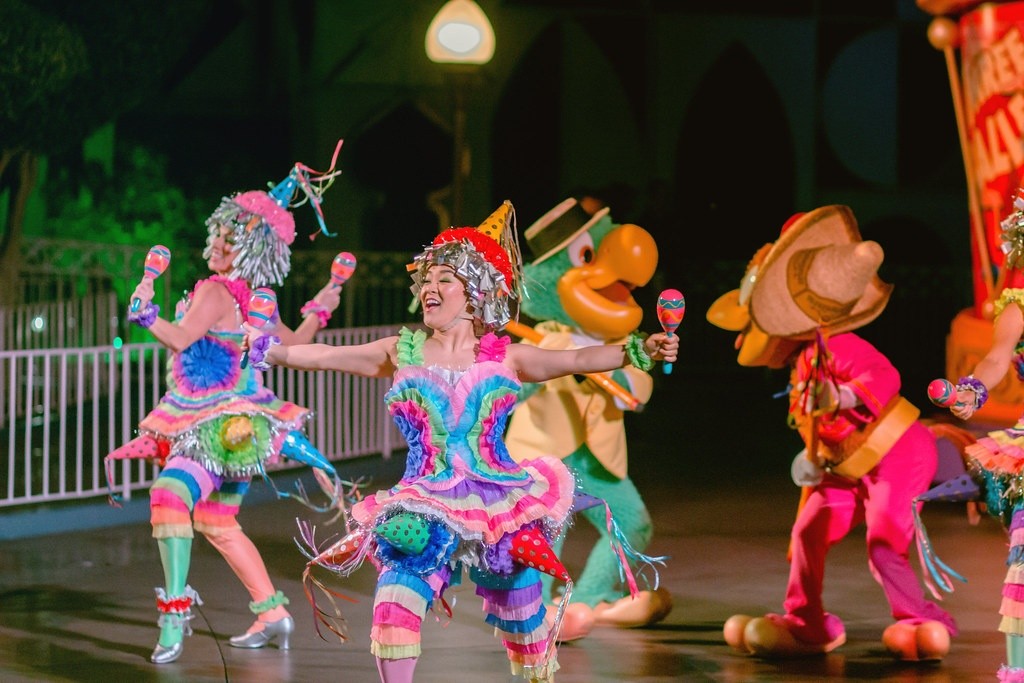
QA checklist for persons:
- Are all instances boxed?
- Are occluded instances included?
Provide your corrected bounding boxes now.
[949,189,1023,683]
[103,166,343,665]
[239,200,680,683]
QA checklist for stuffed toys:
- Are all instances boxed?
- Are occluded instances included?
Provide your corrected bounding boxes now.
[506,199,673,642]
[706,204,963,661]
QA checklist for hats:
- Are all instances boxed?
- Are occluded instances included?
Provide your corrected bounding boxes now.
[230,166,301,245]
[434,200,511,296]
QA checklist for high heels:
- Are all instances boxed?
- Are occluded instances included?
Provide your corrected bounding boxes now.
[229,617,295,651]
[151,610,195,663]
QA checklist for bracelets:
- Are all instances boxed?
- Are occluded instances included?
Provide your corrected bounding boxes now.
[956,376,989,409]
[127,302,160,328]
[301,297,332,327]
[248,334,282,372]
[624,332,656,372]
